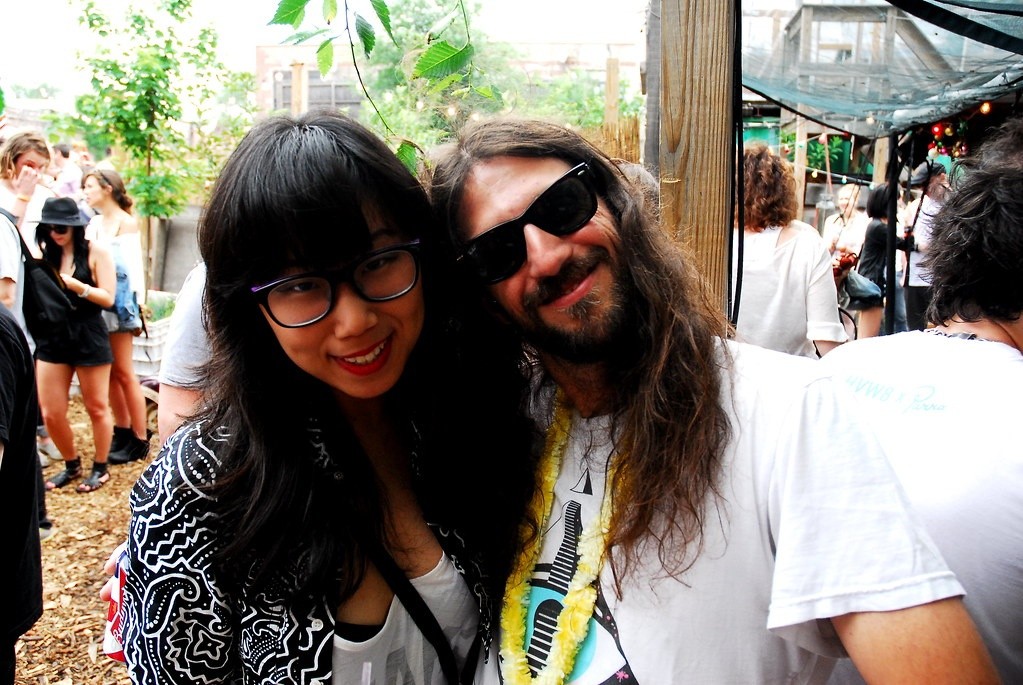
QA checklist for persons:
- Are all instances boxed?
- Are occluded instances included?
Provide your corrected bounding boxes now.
[0,132,153,493]
[157,261,220,448]
[123,105,544,685]
[0,299,48,685]
[819,121,1023,685]
[99,117,1001,685]
[726,139,953,360]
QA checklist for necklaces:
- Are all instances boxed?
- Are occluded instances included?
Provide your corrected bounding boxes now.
[499,388,635,685]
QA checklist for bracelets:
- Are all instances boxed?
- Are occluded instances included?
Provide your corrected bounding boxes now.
[78,284,91,298]
[17,194,31,202]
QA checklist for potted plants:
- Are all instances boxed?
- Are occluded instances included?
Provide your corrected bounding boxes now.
[44,0,263,384]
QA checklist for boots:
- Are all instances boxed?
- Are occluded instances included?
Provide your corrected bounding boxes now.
[44,456,82,490]
[78,462,110,491]
[111,425,134,453]
[109,426,153,465]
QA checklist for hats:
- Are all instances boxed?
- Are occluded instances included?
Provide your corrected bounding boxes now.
[37,197,88,226]
[910,161,945,184]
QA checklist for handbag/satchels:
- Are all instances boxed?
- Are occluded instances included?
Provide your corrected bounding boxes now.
[842,272,881,310]
[25,257,77,335]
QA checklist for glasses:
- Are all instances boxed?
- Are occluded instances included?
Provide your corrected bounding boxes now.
[916,176,938,189]
[43,225,67,235]
[448,161,599,284]
[249,240,420,328]
